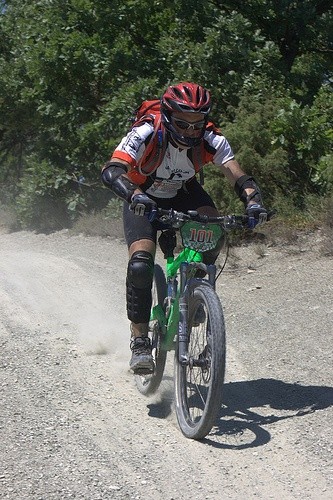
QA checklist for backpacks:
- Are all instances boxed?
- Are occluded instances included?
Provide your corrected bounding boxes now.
[128,100,223,186]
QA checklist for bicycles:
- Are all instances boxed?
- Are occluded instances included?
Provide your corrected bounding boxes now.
[128,201,278,440]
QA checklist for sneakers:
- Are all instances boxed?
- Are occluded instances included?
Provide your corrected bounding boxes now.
[192,304,207,328]
[129,334,156,376]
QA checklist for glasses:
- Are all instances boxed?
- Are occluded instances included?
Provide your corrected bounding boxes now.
[169,116,206,131]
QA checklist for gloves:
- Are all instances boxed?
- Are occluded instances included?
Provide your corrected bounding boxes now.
[128,194,158,222]
[246,203,269,232]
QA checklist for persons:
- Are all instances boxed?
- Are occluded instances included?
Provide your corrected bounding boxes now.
[100,81,268,371]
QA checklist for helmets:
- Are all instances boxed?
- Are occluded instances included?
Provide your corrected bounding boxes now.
[159,82,211,150]
